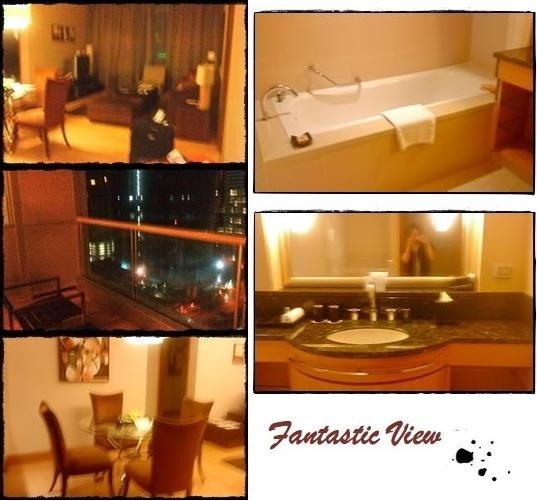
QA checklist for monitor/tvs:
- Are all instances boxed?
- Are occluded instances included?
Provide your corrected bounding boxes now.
[74,53,93,79]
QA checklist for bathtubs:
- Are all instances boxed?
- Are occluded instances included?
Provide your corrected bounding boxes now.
[255,61,496,192]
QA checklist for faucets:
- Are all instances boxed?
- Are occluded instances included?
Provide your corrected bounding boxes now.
[258,84,299,121]
[363,280,379,321]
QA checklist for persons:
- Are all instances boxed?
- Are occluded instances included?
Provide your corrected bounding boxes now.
[402,224,436,276]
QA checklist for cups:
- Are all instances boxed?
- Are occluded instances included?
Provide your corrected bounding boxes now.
[311,304,340,322]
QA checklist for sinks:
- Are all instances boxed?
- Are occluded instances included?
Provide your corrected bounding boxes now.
[324,325,411,345]
